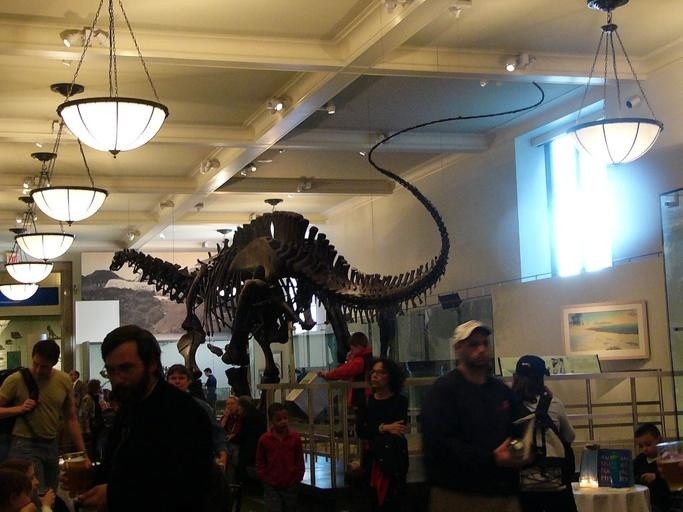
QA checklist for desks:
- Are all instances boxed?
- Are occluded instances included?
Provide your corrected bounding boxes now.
[570,482,652,511]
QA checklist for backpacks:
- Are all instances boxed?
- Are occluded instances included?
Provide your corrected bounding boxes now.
[519,389,575,493]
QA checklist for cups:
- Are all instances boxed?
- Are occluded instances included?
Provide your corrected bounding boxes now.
[55,451,89,495]
[35,487,50,504]
[655,440,683,490]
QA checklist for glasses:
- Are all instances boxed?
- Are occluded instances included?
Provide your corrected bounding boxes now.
[100,366,136,378]
[369,368,385,376]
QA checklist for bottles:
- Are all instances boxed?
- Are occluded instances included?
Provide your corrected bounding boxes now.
[509,439,524,458]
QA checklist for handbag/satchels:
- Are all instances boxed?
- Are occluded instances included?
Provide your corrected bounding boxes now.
[0,367,38,433]
[376,440,408,477]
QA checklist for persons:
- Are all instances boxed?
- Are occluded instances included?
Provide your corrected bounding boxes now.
[501,355,577,512]
[621,421,683,512]
[316,330,373,438]
[55,322,219,512]
[0,338,93,494]
[419,319,554,512]
[1,358,419,512]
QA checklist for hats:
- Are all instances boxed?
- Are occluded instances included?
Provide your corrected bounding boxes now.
[452,320,491,344]
[516,356,545,377]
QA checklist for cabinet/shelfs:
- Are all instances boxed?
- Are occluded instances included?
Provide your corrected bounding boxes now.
[258,382,373,490]
[407,368,683,473]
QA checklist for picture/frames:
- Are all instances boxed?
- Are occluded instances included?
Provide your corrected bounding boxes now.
[561,299,650,361]
[273,351,284,379]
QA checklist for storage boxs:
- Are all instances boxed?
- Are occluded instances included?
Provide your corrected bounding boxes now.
[284,371,337,419]
